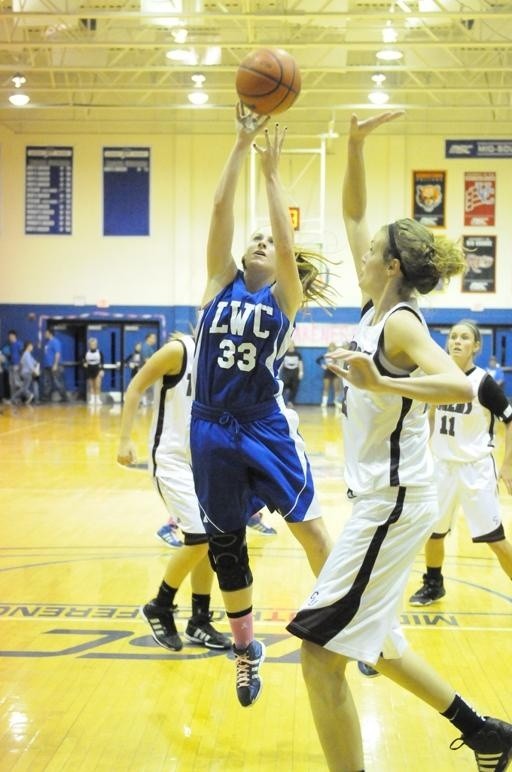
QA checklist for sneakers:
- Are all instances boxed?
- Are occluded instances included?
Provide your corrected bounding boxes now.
[0,393,103,407]
[408,575,447,607]
[357,661,378,676]
[317,400,343,410]
[140,599,230,651]
[233,640,265,708]
[462,716,511,771]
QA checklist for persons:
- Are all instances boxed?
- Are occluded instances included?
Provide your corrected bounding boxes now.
[0,323,158,408]
[406,319,512,607]
[115,328,233,652]
[188,103,381,708]
[283,105,512,772]
[484,356,505,392]
[279,340,304,410]
[314,342,343,408]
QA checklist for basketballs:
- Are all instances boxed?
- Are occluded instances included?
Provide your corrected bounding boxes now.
[237,47,300,114]
